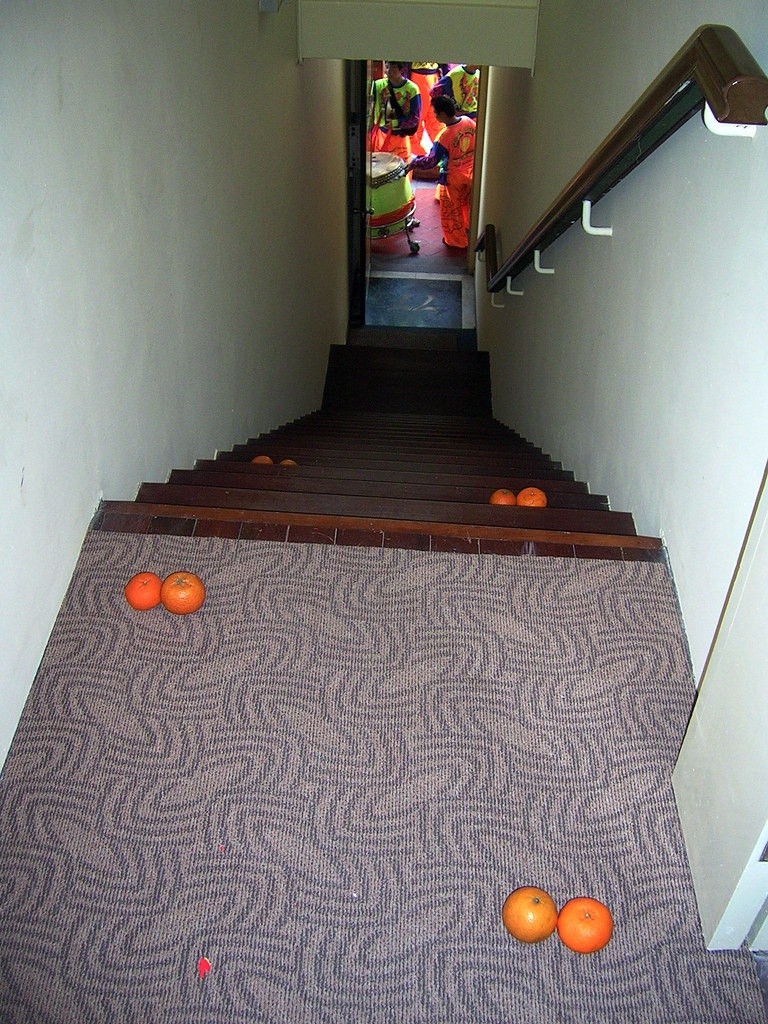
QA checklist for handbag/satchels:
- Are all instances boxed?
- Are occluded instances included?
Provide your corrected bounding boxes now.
[398,113,419,137]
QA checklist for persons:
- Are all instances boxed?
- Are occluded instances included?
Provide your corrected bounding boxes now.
[371,60,421,227]
[405,95,477,255]
[429,64,482,123]
[402,62,452,157]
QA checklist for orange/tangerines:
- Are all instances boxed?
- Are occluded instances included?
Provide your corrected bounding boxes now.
[502,886,558,943]
[160,572,206,615]
[489,488,516,505]
[124,572,163,611]
[279,460,297,464]
[251,456,273,464]
[516,487,547,507]
[557,897,613,953]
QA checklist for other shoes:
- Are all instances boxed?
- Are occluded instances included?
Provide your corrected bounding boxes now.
[412,217,420,226]
[442,237,468,249]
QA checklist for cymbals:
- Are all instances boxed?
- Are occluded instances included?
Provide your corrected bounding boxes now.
[380,98,396,125]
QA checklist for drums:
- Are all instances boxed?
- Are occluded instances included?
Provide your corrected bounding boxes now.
[370,151,417,239]
